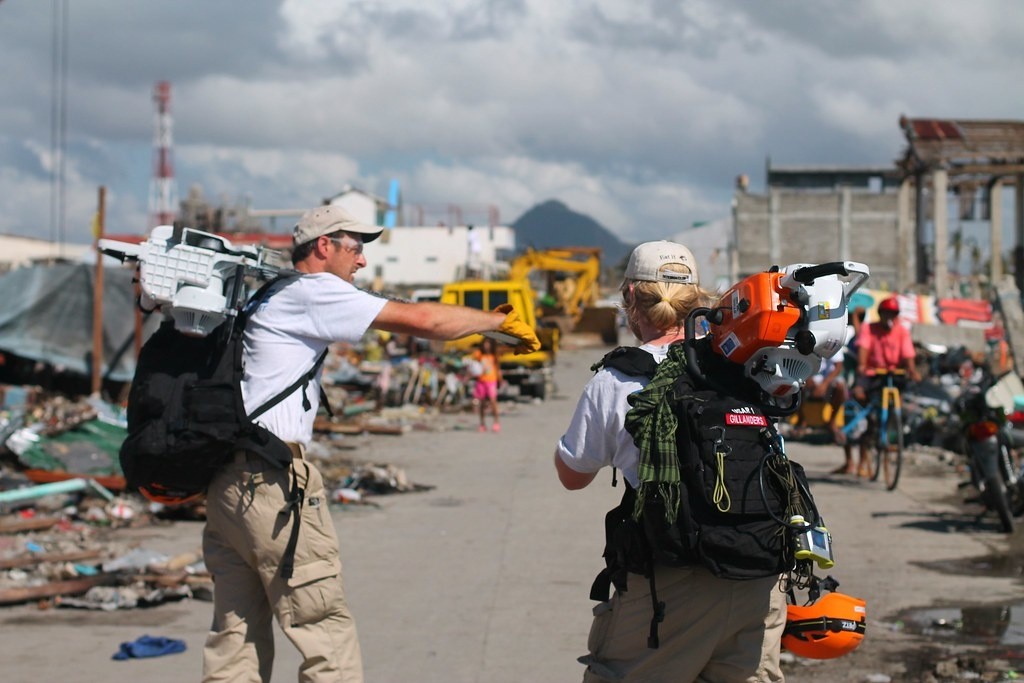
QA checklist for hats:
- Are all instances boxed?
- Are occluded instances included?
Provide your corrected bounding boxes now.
[619,240,700,289]
[294,206,384,246]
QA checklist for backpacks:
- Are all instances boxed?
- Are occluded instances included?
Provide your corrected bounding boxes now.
[590,346,810,602]
[120,269,329,505]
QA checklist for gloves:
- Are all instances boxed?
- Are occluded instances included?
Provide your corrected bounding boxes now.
[493,304,541,355]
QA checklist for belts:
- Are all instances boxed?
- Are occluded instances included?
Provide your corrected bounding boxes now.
[228,443,302,462]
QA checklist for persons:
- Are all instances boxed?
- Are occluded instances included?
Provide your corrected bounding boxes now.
[555,238,786,683]
[804,297,1024,474]
[202,204,543,683]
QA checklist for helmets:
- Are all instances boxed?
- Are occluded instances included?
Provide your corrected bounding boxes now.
[781,593,867,660]
[880,298,898,310]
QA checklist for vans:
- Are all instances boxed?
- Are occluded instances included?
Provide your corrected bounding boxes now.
[410,289,442,352]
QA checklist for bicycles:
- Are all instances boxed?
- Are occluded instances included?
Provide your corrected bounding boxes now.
[864,369,914,492]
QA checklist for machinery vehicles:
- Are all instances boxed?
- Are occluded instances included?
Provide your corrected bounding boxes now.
[432,246,600,397]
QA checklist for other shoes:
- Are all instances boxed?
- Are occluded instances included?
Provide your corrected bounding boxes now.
[479,424,500,432]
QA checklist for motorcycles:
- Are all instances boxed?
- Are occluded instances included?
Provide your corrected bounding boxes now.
[950,386,1024,534]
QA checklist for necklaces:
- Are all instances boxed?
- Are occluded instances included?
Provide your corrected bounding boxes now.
[664,330,684,335]
